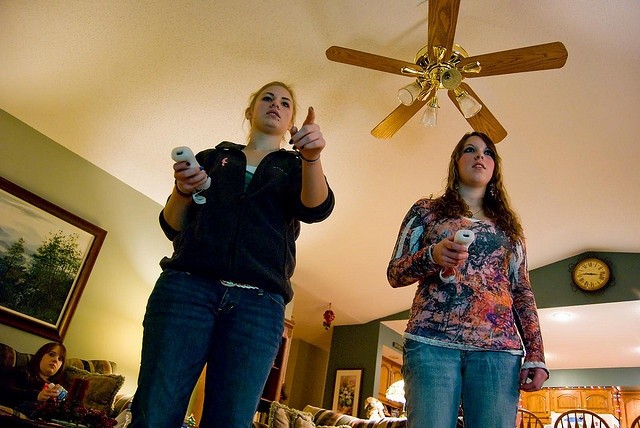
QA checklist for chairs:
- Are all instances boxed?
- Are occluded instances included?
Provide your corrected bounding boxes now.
[517,407,544,428]
[555,410,610,428]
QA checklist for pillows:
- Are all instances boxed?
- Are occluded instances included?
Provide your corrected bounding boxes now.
[289,408,319,428]
[268,400,292,428]
[57,366,124,421]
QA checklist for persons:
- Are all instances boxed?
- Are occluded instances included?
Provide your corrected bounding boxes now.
[386,131,550,426]
[6,341,70,421]
[129,80,337,428]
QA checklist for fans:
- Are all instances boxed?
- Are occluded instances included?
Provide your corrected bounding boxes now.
[325,1,568,147]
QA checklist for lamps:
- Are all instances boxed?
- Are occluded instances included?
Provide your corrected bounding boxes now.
[396,43,481,129]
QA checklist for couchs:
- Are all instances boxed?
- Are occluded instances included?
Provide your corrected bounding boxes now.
[1,340,135,427]
[249,403,408,428]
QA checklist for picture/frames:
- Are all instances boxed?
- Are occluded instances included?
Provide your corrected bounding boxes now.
[1,175,108,343]
[331,365,365,417]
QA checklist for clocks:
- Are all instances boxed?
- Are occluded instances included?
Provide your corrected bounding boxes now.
[568,252,616,296]
